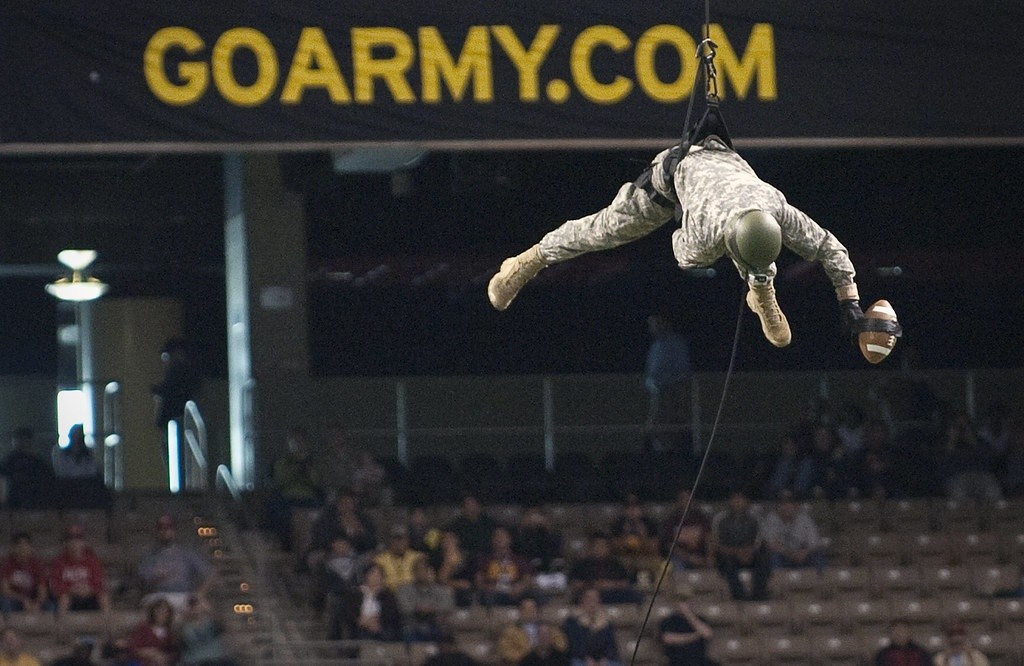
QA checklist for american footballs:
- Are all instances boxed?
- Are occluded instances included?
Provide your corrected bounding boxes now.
[859,299,900,365]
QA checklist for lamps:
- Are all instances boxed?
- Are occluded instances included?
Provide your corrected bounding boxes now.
[47,247,111,303]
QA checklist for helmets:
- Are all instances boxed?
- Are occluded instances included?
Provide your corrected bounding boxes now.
[724,209,782,272]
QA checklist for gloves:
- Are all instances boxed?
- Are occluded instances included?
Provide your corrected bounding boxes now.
[840,300,864,324]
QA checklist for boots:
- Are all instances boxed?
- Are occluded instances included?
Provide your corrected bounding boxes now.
[488,243,549,311]
[746,278,792,347]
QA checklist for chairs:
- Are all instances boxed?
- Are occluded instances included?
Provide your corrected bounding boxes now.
[0,507,184,653]
[295,493,1024,666]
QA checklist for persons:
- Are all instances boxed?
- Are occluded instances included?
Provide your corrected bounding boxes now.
[485,142,864,347]
[0,336,1024,666]
